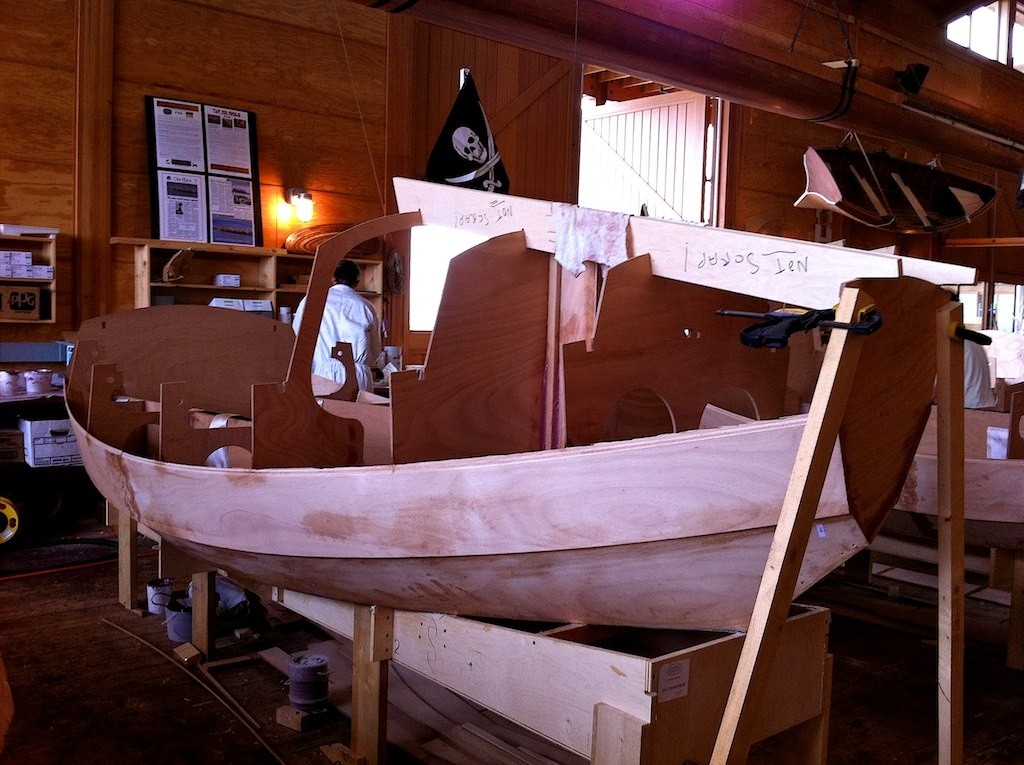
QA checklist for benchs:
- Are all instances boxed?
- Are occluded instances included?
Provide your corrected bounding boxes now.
[849,163,888,217]
[891,173,931,227]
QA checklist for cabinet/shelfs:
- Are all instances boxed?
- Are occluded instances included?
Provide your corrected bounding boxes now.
[109,236,384,348]
[0,235,57,324]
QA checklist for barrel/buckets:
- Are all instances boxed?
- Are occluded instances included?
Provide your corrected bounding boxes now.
[147,576,193,642]
[288,655,333,711]
[24,369,53,394]
[0,369,20,396]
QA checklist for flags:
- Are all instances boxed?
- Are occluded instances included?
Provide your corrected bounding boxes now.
[424,72,510,195]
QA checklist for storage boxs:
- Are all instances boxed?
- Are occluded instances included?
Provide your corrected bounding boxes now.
[0,286,40,320]
[215,274,240,287]
[0,251,54,280]
[0,430,25,464]
[207,297,274,319]
[18,417,84,468]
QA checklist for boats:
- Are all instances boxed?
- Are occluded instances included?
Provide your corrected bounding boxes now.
[877,404,1024,588]
[62,207,954,633]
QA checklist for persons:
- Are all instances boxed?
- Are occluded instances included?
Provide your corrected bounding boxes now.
[963,339,996,408]
[291,262,382,393]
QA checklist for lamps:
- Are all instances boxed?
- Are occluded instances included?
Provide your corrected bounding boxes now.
[283,186,314,223]
[895,63,930,94]
